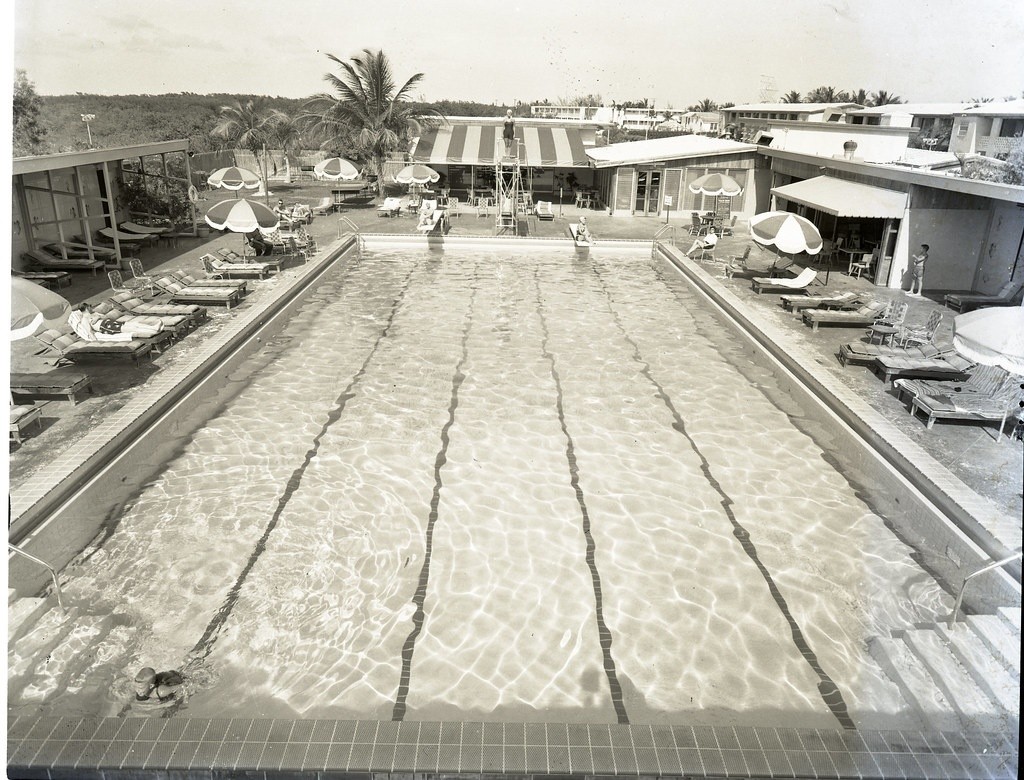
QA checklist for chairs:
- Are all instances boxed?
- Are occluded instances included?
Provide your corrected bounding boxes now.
[871,300,909,345]
[838,340,957,368]
[725,256,794,280]
[802,297,889,332]
[750,264,823,297]
[694,240,718,264]
[875,354,979,384]
[11,197,336,445]
[900,308,943,351]
[908,373,1024,431]
[812,237,880,281]
[728,245,751,264]
[572,186,601,210]
[943,281,1024,313]
[377,188,556,224]
[894,363,1010,412]
[779,289,866,315]
[688,212,738,239]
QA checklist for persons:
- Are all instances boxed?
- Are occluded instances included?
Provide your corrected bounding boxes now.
[501,109,515,160]
[77,302,164,337]
[905,244,929,297]
[575,215,596,244]
[271,199,298,224]
[684,226,718,257]
[420,202,434,227]
[134,667,188,703]
[246,228,274,258]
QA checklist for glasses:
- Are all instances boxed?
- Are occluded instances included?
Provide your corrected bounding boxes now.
[279,203,283,204]
[584,217,586,219]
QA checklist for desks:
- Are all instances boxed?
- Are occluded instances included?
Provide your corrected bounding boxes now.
[840,248,871,277]
[865,240,881,267]
[583,192,593,208]
[700,215,723,235]
[868,325,900,346]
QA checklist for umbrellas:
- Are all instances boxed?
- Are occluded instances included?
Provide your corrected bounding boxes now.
[748,210,824,278]
[689,173,744,216]
[951,305,1024,445]
[204,199,281,265]
[11,273,72,407]
[313,157,363,213]
[207,160,262,199]
[395,164,440,215]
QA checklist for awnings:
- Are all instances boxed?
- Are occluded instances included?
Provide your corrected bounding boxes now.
[770,174,908,219]
[405,125,590,168]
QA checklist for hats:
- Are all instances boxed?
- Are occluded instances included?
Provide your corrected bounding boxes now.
[579,217,586,221]
